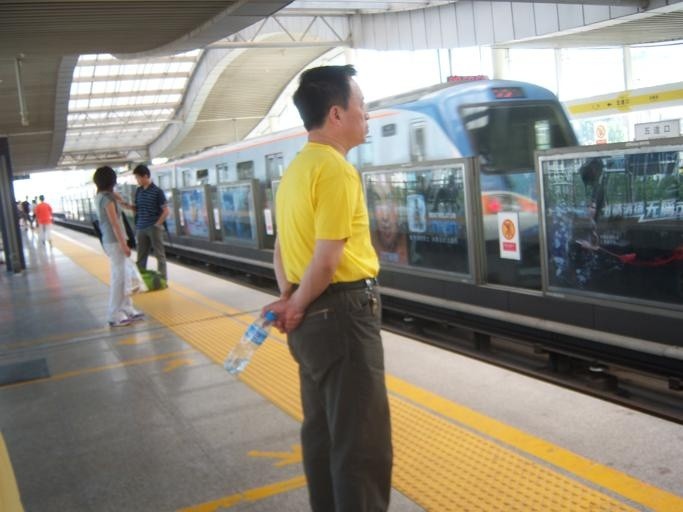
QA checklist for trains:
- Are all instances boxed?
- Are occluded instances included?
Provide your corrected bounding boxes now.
[59,72,599,291]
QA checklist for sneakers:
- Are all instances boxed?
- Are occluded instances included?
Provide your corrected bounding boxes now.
[105,308,146,327]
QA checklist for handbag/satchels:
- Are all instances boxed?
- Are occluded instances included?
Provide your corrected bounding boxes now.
[91,211,136,256]
[137,269,167,292]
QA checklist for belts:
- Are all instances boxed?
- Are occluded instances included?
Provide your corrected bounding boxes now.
[290,276,378,295]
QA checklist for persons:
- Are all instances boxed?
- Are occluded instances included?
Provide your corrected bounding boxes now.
[429,170,462,213]
[364,172,409,266]
[256,62,393,512]
[562,197,600,266]
[573,156,605,222]
[31,194,39,229]
[90,164,145,330]
[412,197,424,230]
[20,195,34,231]
[35,195,54,249]
[117,161,171,290]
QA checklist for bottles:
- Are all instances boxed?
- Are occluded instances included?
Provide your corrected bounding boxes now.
[223,310,277,379]
